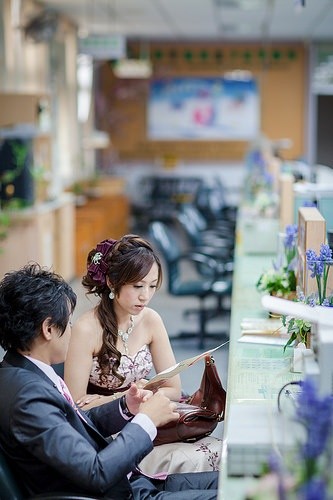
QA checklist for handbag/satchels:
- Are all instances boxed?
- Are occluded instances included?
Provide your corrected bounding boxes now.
[153,354,225,446]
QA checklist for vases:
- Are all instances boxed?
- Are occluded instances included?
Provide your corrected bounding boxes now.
[305,332,311,349]
[291,345,310,374]
[269,291,296,319]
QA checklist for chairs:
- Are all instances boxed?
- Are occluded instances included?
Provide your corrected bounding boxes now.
[0,464,100,500]
[130,178,238,349]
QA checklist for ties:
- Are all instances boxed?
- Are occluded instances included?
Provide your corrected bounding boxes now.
[59,379,168,480]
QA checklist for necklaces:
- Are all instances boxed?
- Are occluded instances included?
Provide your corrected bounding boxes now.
[116,314,134,353]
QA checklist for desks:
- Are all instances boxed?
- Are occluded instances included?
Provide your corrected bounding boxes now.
[216,185,333,500]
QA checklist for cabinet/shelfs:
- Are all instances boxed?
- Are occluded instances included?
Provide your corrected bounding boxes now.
[1,194,82,284]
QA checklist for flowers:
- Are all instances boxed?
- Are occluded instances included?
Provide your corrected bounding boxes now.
[296,243,333,307]
[255,226,300,293]
[275,311,310,349]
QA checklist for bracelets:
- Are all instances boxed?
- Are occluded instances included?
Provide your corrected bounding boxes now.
[111,391,123,400]
[119,395,134,418]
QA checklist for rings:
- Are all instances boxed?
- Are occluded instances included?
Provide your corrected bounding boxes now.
[98,395,102,399]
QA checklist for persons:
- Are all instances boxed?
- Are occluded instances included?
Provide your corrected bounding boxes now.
[0,259,220,500]
[61,235,224,480]
